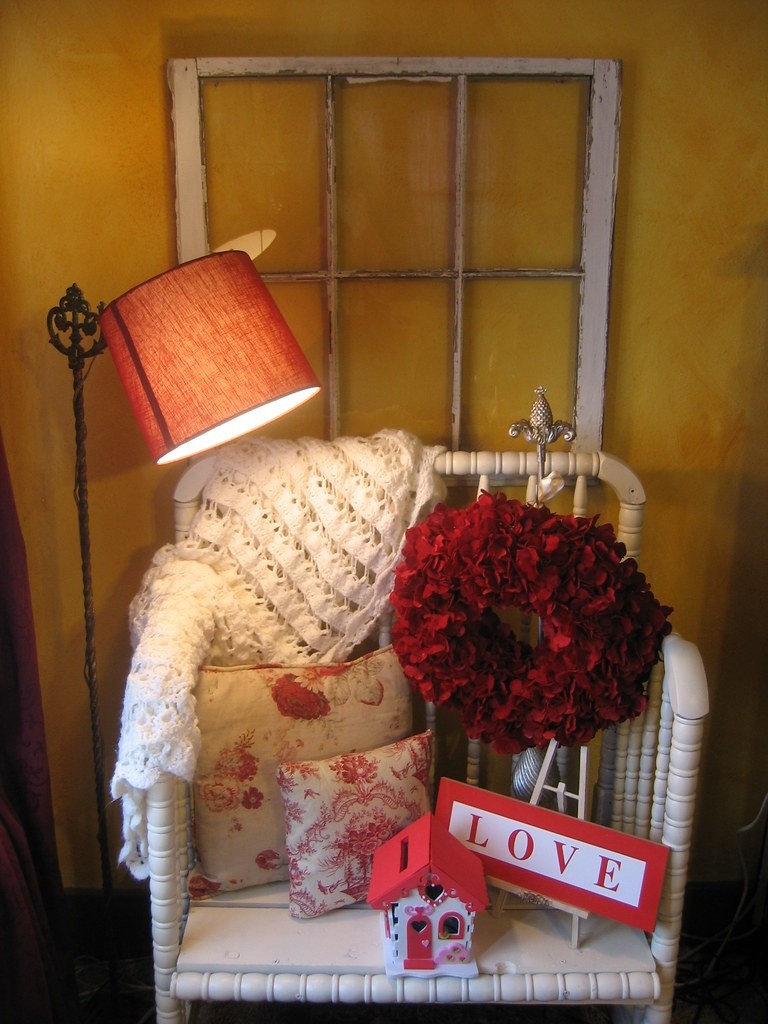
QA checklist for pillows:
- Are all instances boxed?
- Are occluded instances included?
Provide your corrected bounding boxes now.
[189,646,433,921]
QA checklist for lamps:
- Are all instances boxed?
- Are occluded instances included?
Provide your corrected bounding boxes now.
[47,250,322,1024]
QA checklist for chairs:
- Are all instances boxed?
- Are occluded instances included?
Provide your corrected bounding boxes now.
[110,429,709,1024]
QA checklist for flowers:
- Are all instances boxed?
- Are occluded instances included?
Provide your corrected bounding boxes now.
[391,488,674,754]
